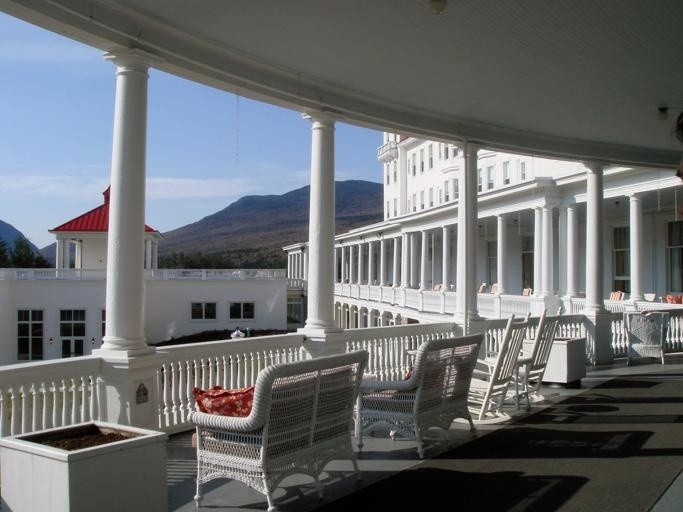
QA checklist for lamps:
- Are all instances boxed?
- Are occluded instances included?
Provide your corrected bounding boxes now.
[656,107,672,121]
